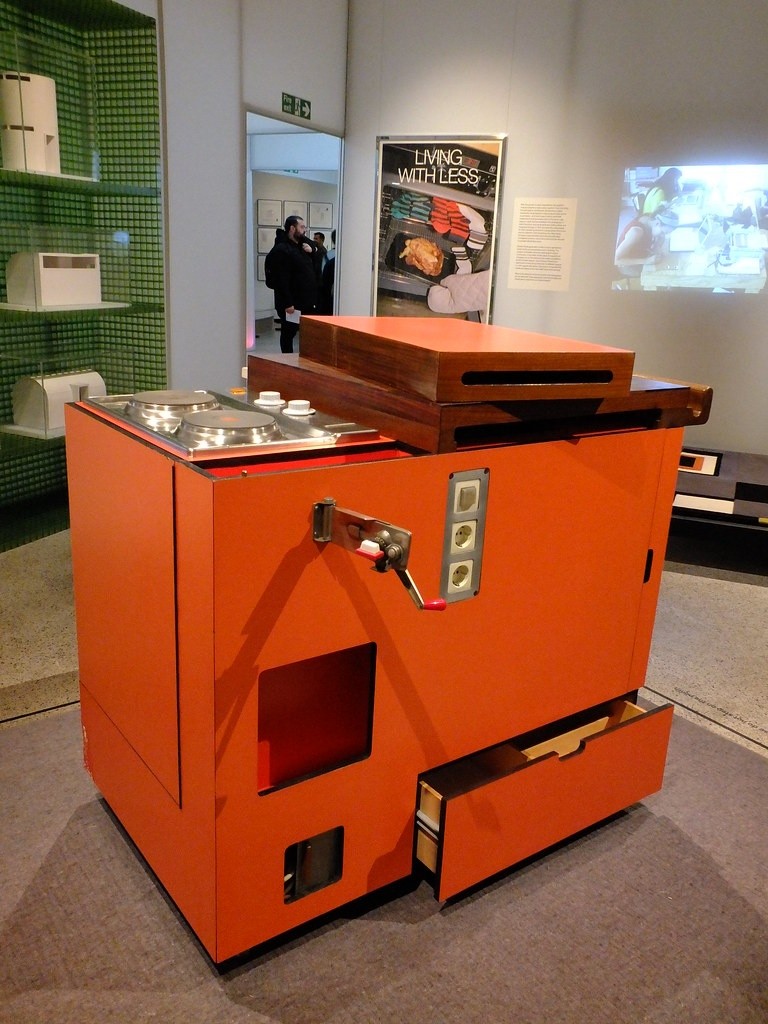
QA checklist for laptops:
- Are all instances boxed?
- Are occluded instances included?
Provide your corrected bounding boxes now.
[670,216,712,251]
[723,219,768,248]
[680,193,702,205]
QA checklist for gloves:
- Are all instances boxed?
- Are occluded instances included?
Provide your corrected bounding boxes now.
[427,269,490,314]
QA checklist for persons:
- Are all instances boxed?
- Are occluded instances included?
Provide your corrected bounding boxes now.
[613,205,678,290]
[274,215,320,353]
[642,168,683,215]
[312,228,337,316]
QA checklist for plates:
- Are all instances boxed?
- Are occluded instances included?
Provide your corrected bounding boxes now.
[386,232,457,287]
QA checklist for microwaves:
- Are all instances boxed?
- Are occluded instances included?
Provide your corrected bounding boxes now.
[378,172,494,297]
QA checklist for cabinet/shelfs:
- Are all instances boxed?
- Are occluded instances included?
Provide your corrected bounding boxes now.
[0,0,168,722]
